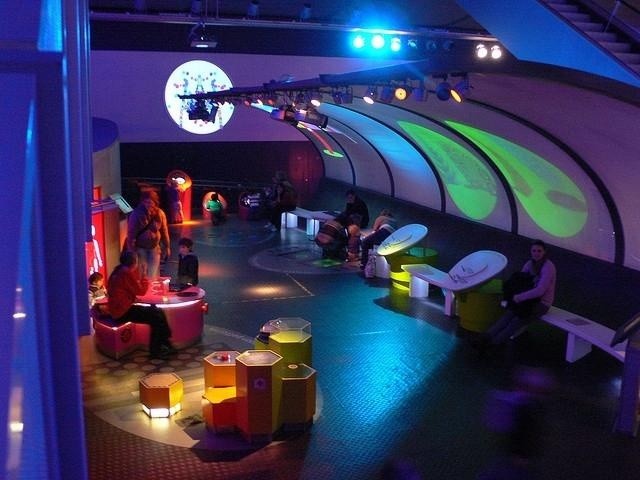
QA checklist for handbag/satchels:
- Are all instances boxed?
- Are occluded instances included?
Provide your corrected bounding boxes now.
[507,271,534,293]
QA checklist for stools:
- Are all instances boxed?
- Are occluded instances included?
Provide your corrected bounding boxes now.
[89,271,318,445]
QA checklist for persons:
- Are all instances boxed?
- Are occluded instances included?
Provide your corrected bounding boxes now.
[471,240,556,352]
[165,179,187,223]
[315,211,352,260]
[246,169,295,233]
[108,250,178,359]
[86,272,108,317]
[177,236,199,287]
[206,192,227,226]
[127,188,171,275]
[346,224,361,263]
[361,209,397,268]
[345,189,370,228]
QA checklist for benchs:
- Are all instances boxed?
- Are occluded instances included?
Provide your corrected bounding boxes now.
[280,206,336,236]
[357,227,627,367]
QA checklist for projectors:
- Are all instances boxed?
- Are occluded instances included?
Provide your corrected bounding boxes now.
[191,35,217,49]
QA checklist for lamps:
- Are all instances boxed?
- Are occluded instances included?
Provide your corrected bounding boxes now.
[189,99,220,123]
[362,80,378,104]
[270,107,329,129]
[379,80,396,104]
[411,76,429,102]
[351,26,503,62]
[219,88,324,107]
[331,86,353,104]
[435,74,455,101]
[394,80,413,101]
[450,71,474,104]
[187,26,219,49]
